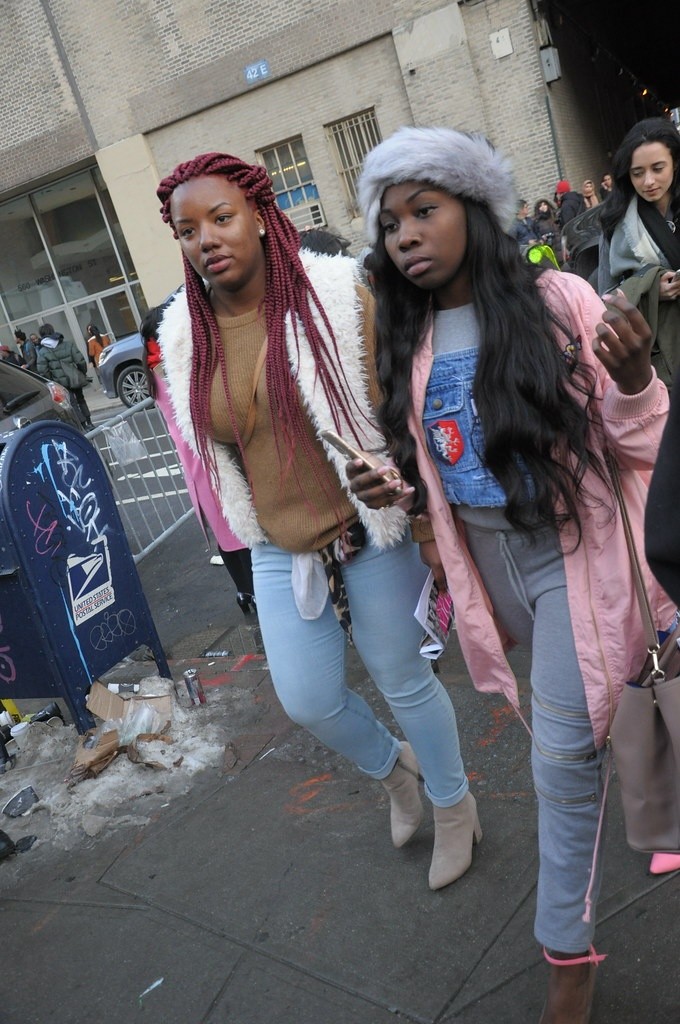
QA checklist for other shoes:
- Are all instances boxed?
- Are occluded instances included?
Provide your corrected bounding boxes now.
[649,852,680,873]
[540,945,595,1024]
[85,424,95,432]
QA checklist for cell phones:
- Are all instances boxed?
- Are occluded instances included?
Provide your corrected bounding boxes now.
[670,269,680,283]
[320,430,403,495]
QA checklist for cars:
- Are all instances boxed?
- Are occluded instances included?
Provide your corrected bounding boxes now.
[560,199,609,291]
[96,332,155,412]
[0,358,114,491]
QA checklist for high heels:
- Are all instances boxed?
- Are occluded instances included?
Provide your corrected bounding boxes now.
[237,592,252,616]
[428,791,482,890]
[251,596,257,615]
[381,740,426,849]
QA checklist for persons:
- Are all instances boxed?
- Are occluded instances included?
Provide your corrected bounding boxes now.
[341,124,671,1023]
[1,321,111,431]
[157,151,484,895]
[137,112,680,645]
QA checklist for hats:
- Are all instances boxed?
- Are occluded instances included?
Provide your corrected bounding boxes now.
[556,181,570,193]
[0,345,12,354]
[358,130,520,268]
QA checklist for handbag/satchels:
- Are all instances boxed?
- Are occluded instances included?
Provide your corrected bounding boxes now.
[61,361,87,390]
[606,624,680,855]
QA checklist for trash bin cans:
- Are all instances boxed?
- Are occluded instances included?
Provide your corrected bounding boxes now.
[0,420,181,750]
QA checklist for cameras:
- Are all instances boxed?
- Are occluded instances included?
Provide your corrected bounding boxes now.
[554,214,561,226]
[545,232,554,247]
